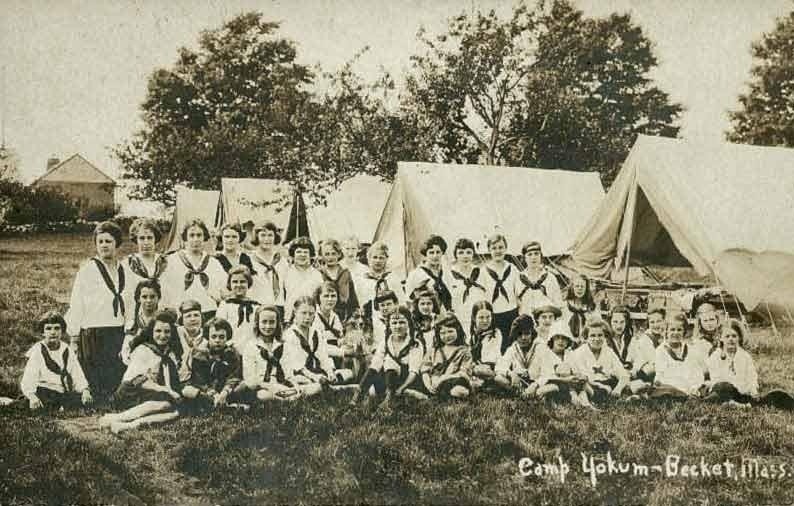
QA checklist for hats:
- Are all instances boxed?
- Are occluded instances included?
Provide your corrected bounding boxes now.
[532,304,562,318]
[699,302,715,312]
[522,241,542,254]
[180,299,202,312]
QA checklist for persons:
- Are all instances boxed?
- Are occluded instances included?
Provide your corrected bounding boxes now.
[0,309,97,413]
[64,217,763,440]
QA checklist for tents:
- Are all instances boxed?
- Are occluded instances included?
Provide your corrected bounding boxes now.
[163,131,793,321]
